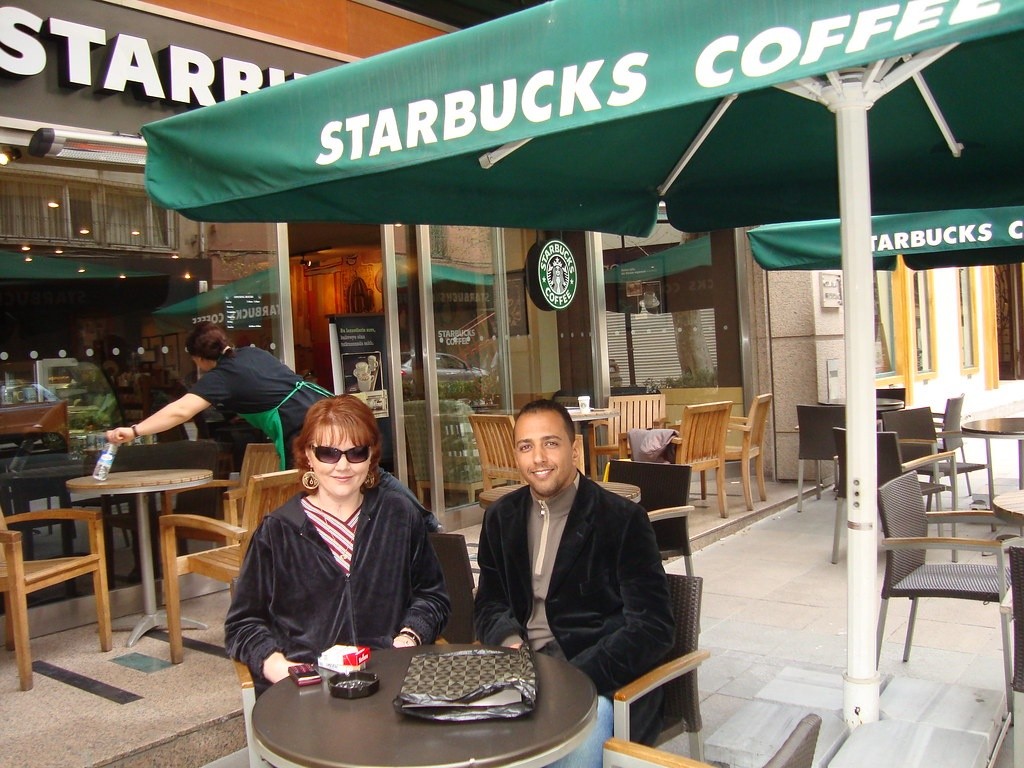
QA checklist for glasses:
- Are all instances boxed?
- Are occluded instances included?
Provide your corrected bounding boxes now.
[309,444,370,464]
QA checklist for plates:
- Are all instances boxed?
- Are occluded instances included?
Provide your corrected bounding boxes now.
[353,369,371,377]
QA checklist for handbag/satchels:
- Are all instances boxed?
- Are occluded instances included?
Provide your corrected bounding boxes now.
[392,643,539,721]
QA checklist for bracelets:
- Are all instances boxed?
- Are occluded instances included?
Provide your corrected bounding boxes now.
[393,631,420,646]
[131,424,140,439]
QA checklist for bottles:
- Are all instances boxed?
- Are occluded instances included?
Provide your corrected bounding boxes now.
[92,442,120,482]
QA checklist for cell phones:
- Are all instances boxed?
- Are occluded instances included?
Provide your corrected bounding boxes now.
[287,664,322,687]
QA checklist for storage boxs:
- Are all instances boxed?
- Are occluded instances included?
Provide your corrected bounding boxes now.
[320,645,371,666]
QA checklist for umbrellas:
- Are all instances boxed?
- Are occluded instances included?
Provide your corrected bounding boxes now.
[140,0,1024,727]
[603,233,714,315]
[396,257,496,313]
[747,205,1024,270]
[0,250,282,324]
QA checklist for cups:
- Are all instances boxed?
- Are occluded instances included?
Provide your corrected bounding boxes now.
[357,373,374,391]
[17,388,38,404]
[368,355,378,372]
[578,396,590,414]
[355,362,368,374]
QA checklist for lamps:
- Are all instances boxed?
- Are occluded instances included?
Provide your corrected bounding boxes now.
[25,126,148,168]
[0,146,23,166]
[300,259,312,266]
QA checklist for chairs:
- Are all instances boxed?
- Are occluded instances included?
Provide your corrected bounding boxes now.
[0,386,1024,768]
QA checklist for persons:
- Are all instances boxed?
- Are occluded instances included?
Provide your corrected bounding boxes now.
[224,395,452,703]
[153,382,247,442]
[106,322,335,472]
[473,400,670,768]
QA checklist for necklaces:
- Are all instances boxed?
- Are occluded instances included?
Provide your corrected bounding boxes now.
[316,492,362,561]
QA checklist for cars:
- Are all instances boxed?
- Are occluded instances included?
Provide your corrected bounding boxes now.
[0,384,67,448]
[401,351,490,382]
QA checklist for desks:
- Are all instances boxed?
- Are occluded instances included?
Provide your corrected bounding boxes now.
[961,418,1024,557]
[991,489,1024,537]
[817,397,906,412]
[251,644,599,768]
[65,468,214,648]
[567,407,622,422]
